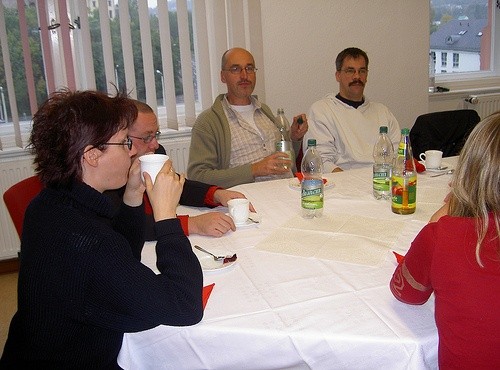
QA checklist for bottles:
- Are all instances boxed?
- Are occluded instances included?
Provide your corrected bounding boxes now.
[373,126,394,200]
[429,53,436,94]
[390,128,417,214]
[300,139,324,219]
[274,108,291,169]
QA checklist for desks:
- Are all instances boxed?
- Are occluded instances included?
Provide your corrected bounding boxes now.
[117,157,457,370]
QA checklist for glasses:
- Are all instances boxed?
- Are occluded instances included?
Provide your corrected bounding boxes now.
[91,134,133,151]
[222,64,259,74]
[126,130,162,144]
[340,68,369,76]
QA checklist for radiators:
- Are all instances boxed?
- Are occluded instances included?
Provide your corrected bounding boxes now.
[464,92,500,121]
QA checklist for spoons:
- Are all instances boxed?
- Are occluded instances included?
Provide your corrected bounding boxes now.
[432,169,455,178]
[194,244,225,261]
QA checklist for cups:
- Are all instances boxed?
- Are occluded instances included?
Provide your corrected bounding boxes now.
[138,154,169,184]
[419,150,443,170]
[227,198,250,224]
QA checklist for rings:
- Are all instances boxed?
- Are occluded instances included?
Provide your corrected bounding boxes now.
[170,170,175,174]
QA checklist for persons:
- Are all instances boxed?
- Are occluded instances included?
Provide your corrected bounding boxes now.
[303,47,402,174]
[1,88,203,370]
[187,47,309,189]
[105,98,257,238]
[390,111,500,370]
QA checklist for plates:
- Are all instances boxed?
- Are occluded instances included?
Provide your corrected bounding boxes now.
[421,162,454,173]
[198,253,238,271]
[288,178,336,192]
[225,212,262,227]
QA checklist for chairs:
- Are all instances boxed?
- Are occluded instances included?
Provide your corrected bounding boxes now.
[410,110,480,161]
[2,174,46,241]
